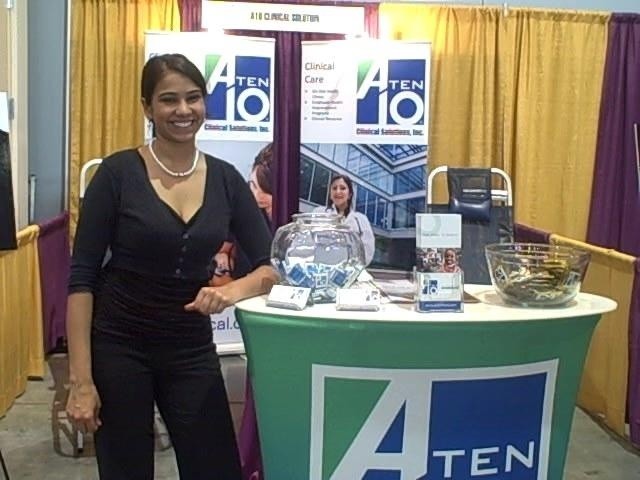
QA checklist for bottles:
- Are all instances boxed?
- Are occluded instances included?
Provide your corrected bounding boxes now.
[271,214,367,305]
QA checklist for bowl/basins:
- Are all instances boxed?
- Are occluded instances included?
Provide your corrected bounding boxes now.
[484,243,591,307]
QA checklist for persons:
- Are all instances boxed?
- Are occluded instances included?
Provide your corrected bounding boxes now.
[63,51,288,480]
[208,140,275,283]
[311,173,377,269]
[440,248,461,274]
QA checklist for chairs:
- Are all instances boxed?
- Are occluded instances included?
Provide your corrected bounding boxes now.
[426,165,515,285]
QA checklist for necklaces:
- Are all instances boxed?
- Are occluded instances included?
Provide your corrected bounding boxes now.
[146,134,202,179]
